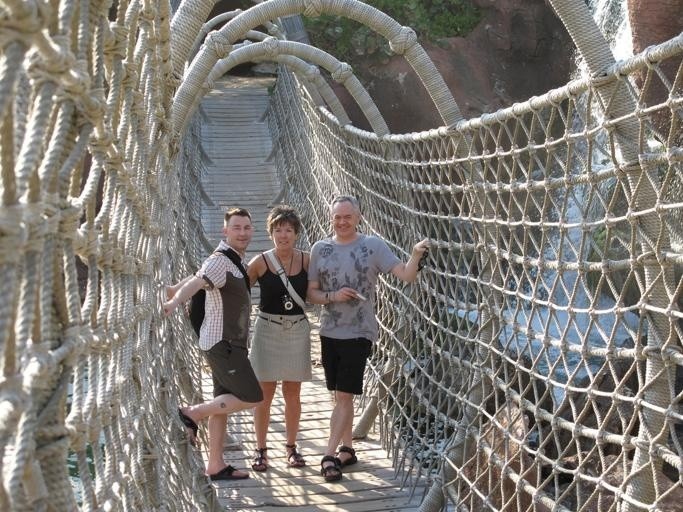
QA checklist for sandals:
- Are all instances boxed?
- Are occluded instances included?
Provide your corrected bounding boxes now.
[253,447,267,471]
[320,456,342,480]
[286,444,305,466]
[336,446,358,469]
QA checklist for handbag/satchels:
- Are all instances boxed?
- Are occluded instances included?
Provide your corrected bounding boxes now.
[190,250,250,337]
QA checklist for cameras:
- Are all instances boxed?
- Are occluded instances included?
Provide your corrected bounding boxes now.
[281,295,294,311]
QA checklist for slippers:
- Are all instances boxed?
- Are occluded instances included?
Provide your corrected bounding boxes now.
[209,465,249,480]
[179,409,198,446]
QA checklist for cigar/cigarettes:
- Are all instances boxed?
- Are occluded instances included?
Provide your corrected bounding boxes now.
[355,291,366,302]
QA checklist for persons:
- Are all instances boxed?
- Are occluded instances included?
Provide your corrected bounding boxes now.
[162,206,265,479]
[307,193,430,482]
[247,205,312,471]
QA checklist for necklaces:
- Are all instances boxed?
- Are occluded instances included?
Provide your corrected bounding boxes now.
[276,249,293,311]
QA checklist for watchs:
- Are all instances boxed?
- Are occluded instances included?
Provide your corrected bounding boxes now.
[323,291,332,304]
